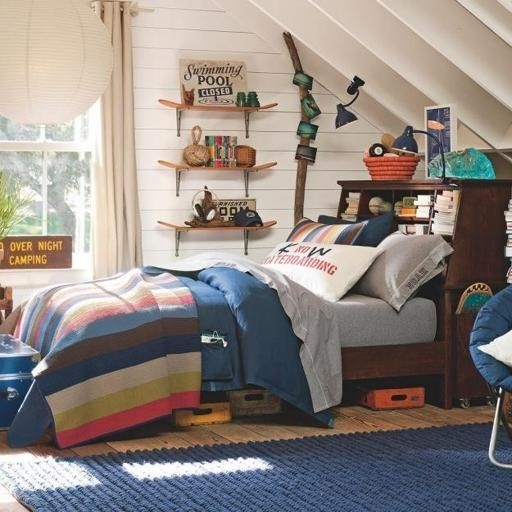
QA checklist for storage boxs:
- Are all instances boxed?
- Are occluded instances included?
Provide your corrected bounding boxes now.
[0,332,41,431]
[225,389,283,416]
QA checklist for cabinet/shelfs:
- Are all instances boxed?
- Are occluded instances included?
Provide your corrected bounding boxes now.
[157,98,278,257]
[336,177,512,408]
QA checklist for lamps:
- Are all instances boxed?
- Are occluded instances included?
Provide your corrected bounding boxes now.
[391,124,445,177]
[335,75,365,129]
[1,0,114,128]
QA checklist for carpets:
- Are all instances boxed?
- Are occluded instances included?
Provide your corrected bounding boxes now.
[0,420,512,512]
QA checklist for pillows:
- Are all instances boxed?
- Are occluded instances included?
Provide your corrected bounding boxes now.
[287,216,369,250]
[373,234,456,311]
[270,241,383,301]
[477,329,512,367]
[319,210,397,247]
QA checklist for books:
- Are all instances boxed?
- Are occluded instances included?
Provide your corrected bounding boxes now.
[397,190,458,236]
[503,199,512,284]
[205,136,238,167]
[340,191,361,222]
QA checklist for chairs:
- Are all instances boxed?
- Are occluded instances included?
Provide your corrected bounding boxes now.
[469,287,512,471]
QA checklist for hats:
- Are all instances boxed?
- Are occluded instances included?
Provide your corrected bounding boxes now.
[233,210,263,227]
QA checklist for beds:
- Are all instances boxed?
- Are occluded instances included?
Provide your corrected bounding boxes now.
[24,266,455,409]
